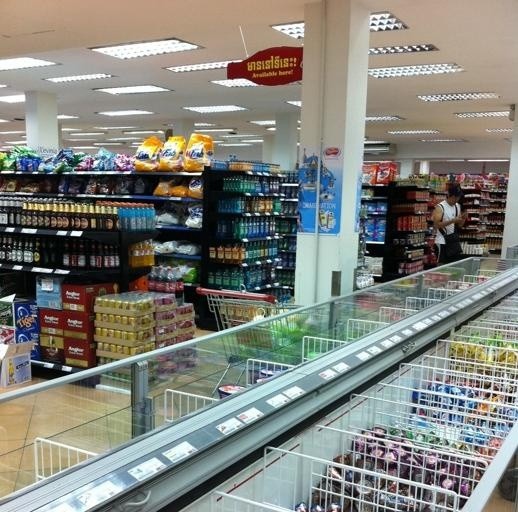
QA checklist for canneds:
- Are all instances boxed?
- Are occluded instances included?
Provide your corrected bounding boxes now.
[94,292,198,383]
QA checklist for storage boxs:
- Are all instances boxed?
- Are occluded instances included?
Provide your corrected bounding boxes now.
[65,338,99,368]
[65,310,95,340]
[1,347,32,387]
[62,282,119,312]
[39,307,65,336]
[1,295,40,330]
[40,335,66,365]
[1,326,39,359]
[35,276,62,310]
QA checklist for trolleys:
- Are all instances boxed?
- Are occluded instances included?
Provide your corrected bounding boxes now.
[197,275,324,398]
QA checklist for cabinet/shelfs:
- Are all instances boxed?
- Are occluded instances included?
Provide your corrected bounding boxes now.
[206,171,284,324]
[2,194,154,296]
[486,189,507,250]
[433,191,489,255]
[395,186,429,280]
[361,183,393,280]
[2,166,206,294]
[279,170,300,302]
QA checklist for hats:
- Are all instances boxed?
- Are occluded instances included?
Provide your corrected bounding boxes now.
[449,183,464,197]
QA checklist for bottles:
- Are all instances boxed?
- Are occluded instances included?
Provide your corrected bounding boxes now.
[208,159,281,291]
[147,266,184,305]
[488,189,506,250]
[0,197,156,273]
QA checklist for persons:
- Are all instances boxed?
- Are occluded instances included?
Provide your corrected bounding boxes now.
[432,183,466,265]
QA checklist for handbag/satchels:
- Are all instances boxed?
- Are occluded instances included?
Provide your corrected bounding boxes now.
[446,233,462,256]
